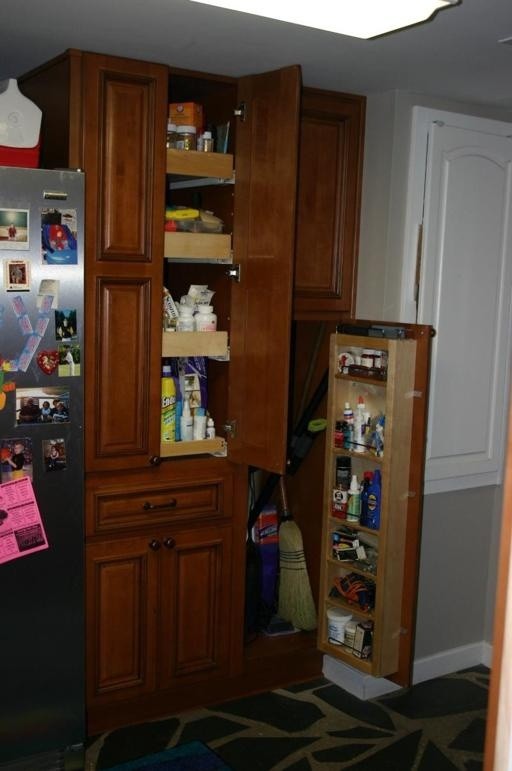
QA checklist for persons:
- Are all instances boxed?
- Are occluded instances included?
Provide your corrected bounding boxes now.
[17,397,69,424]
[48,446,59,468]
[8,223,17,241]
[11,265,22,283]
[59,318,73,339]
[5,440,26,470]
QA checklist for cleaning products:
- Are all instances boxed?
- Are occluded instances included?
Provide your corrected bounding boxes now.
[363,467,382,529]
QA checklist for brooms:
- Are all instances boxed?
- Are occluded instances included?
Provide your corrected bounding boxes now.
[275,475,319,634]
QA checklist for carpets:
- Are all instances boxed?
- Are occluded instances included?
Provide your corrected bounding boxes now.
[102,738,235,771]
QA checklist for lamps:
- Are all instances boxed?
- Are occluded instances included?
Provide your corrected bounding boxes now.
[189,0,463,42]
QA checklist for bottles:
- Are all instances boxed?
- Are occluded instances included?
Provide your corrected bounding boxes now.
[178,303,218,332]
[197,131,214,152]
[331,343,390,531]
[162,366,216,441]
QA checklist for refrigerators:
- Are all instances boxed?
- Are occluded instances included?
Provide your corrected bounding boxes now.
[0,165,84,762]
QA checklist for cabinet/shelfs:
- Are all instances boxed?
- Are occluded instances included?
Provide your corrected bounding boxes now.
[400,104,512,496]
[16,47,436,736]
